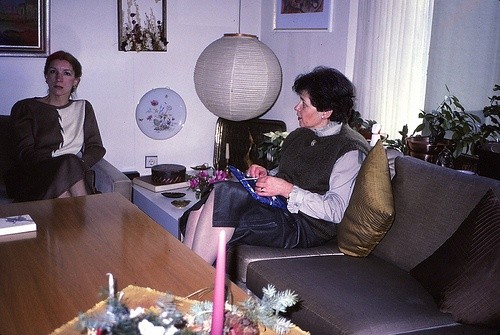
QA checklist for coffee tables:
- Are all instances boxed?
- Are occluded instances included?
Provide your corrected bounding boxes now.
[0,192,308,335]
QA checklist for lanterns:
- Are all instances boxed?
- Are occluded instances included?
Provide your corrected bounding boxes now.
[194,33,283,120]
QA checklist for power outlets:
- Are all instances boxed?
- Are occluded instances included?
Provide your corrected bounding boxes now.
[144,156,158,168]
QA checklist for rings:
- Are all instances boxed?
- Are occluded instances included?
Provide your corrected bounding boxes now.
[261,188,263,192]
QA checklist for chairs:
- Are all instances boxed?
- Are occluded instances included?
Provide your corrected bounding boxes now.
[0,114,132,200]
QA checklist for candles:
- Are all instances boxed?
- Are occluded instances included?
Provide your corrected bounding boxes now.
[105,272,115,299]
[211,230,226,335]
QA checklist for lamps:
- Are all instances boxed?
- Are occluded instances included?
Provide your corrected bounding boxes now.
[193,0,282,121]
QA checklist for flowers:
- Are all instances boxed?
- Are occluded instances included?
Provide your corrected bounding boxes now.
[257,132,290,162]
[190,170,230,198]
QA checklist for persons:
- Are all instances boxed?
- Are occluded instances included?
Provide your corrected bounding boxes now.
[179,66,371,266]
[11,51,106,199]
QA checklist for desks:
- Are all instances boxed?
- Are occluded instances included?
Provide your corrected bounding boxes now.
[132,172,204,241]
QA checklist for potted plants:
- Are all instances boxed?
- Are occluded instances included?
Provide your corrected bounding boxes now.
[407,83,500,181]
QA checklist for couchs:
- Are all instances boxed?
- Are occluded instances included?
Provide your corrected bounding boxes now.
[214,149,500,335]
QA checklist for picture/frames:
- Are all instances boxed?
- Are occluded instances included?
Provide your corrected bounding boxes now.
[272,0,333,34]
[116,0,168,52]
[0,0,50,58]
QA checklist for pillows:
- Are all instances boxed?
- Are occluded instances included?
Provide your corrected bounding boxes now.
[409,188,500,328]
[336,139,395,257]
